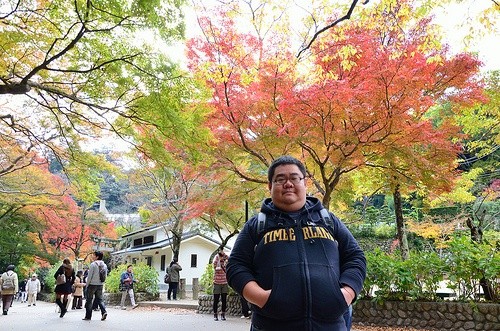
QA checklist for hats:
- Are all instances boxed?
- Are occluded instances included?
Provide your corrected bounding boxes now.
[32,274,36,277]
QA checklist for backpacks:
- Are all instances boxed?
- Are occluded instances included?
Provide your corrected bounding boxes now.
[1,272,15,290]
[92,261,106,283]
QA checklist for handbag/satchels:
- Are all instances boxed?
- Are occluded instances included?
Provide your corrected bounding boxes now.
[164,275,170,283]
[56,266,66,285]
[72,285,77,293]
[119,283,127,292]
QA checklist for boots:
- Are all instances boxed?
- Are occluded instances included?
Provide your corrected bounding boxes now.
[62,299,68,308]
[55,298,67,318]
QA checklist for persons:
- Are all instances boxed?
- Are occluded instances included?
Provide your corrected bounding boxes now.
[54,266,106,313]
[120,265,139,310]
[54,258,76,318]
[225,155,366,331]
[240,294,252,319]
[82,251,107,321]
[165,258,182,300]
[212,246,229,321]
[0,264,42,315]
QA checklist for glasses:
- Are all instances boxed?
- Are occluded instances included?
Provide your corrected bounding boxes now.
[271,176,305,185]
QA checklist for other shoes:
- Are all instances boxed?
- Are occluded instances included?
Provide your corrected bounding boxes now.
[213,312,218,320]
[221,313,226,320]
[101,312,107,320]
[121,307,126,310]
[28,304,36,307]
[132,304,139,309]
[76,306,82,309]
[72,307,75,310]
[82,318,91,320]
[3,308,7,315]
